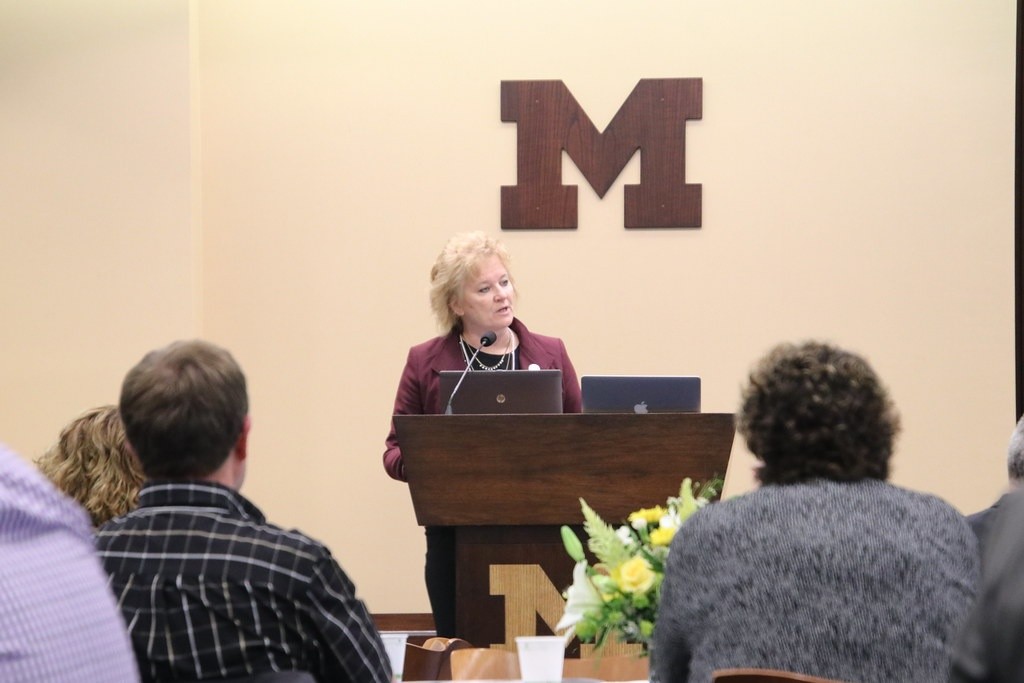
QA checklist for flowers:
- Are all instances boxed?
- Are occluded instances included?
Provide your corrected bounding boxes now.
[553,476,724,672]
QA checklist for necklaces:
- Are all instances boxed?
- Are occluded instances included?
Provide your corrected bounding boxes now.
[459,328,516,370]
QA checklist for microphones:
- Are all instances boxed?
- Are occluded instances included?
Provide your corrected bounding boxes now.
[445,331,497,415]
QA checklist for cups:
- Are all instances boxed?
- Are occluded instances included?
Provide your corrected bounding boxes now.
[514,635,565,683]
[380,633,408,683]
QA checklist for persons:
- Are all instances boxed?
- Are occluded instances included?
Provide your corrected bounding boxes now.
[0,339,398,683]
[648,339,1024,683]
[383,231,583,637]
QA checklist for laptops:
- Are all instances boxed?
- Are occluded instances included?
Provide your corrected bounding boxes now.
[440,371,563,414]
[580,376,702,412]
